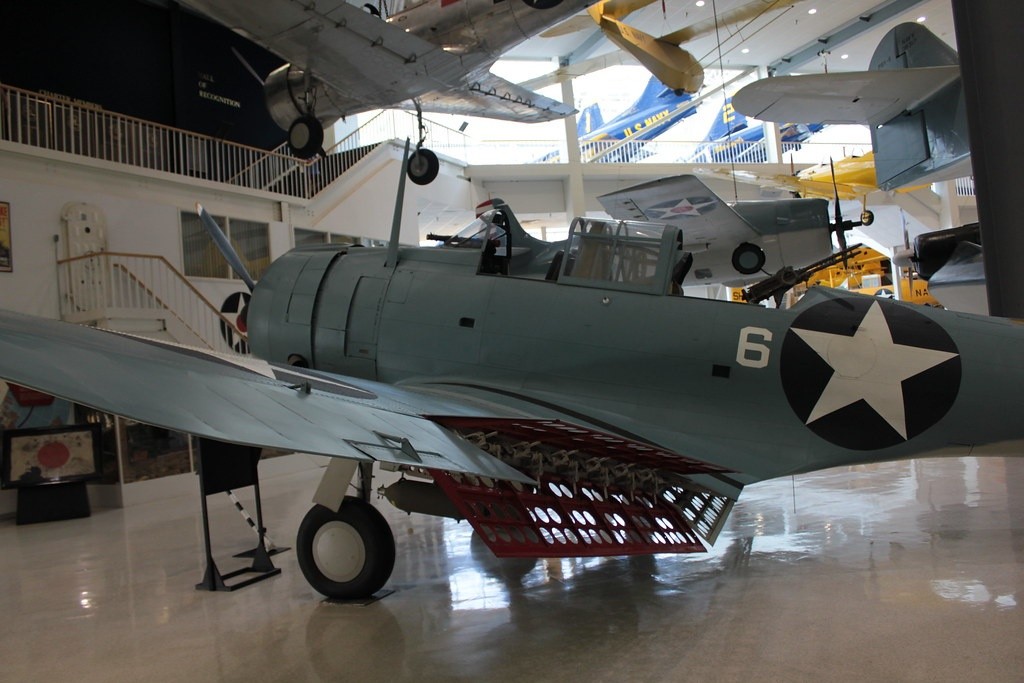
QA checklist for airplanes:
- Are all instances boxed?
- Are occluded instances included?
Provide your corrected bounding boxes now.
[0,2,1022,604]
